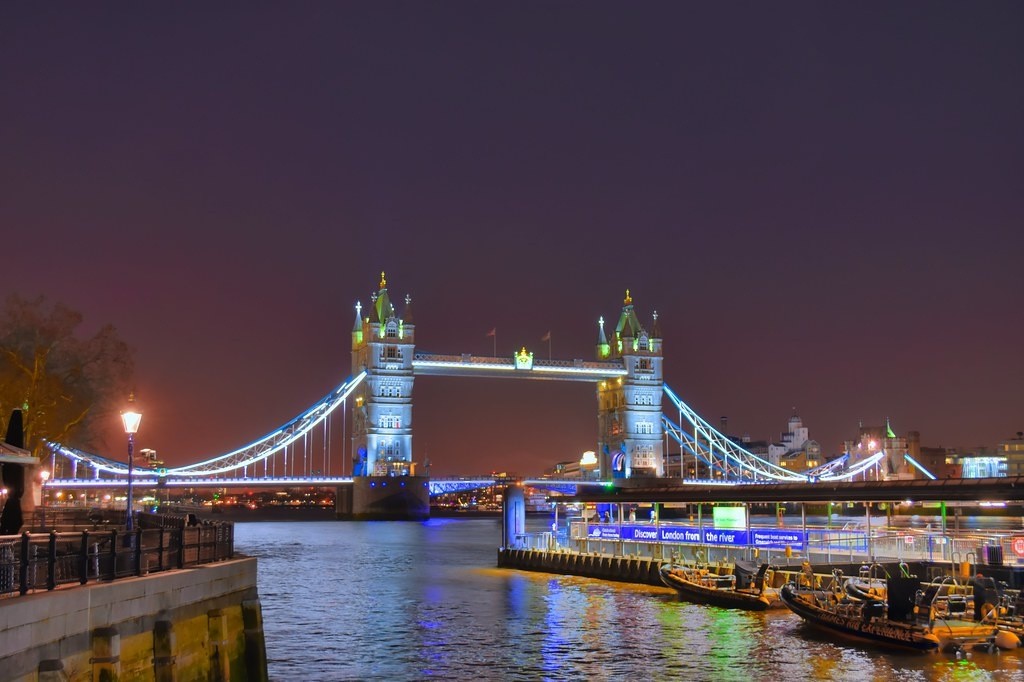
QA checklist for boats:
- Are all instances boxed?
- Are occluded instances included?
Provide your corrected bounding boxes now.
[849,564,1024,644]
[657,559,788,610]
[779,563,998,658]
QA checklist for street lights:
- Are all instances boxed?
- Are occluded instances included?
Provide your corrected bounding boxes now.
[120,388,144,546]
[42,470,50,532]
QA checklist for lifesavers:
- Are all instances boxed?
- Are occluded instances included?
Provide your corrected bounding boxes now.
[980,603,997,620]
